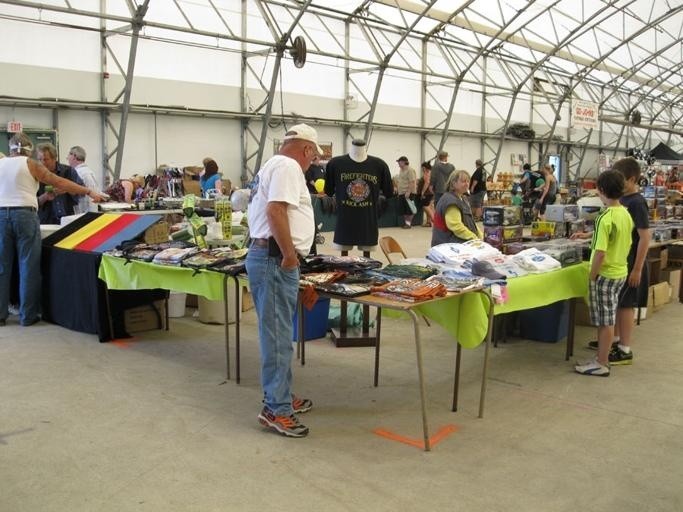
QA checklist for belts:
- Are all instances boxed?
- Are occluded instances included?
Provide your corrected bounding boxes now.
[251,239,268,247]
[0,206,36,211]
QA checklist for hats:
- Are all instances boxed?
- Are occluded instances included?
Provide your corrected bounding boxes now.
[288,125,324,155]
[472,261,507,281]
[396,157,408,161]
[439,152,449,156]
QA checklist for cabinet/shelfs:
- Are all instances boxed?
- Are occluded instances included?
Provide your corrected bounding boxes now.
[484,169,524,208]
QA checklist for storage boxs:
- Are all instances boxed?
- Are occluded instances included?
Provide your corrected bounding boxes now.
[121,162,232,210]
[645,242,682,314]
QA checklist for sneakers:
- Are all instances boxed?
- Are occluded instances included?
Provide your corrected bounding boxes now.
[290,394,312,413]
[21,315,41,326]
[258,405,309,437]
[588,339,620,350]
[574,357,610,377]
[608,347,633,366]
[402,222,431,228]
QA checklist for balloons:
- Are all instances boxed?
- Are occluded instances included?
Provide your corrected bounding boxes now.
[313,178,324,192]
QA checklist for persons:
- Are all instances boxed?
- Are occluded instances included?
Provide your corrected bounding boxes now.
[304,156,326,193]
[428,169,482,248]
[198,157,212,178]
[323,137,396,251]
[102,174,145,205]
[654,166,682,186]
[0,130,107,329]
[244,123,330,440]
[571,167,634,378]
[569,156,657,366]
[199,160,224,199]
[392,147,560,229]
